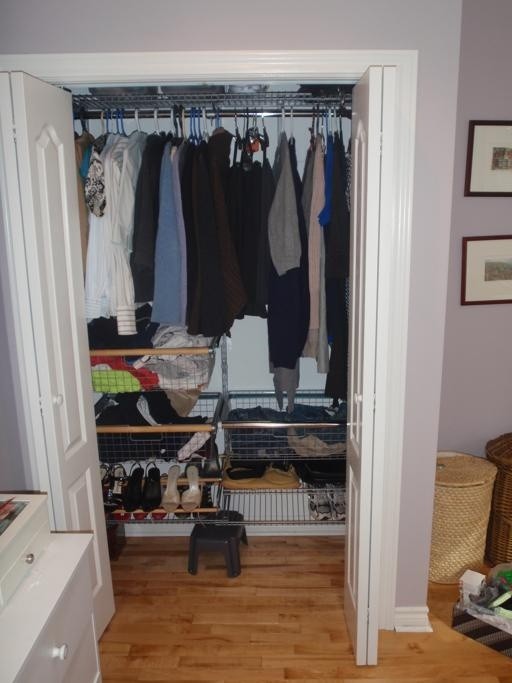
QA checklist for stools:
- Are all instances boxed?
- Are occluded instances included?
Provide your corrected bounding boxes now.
[186,511,249,579]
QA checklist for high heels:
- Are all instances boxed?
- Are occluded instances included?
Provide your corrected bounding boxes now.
[161,462,180,513]
[104,462,127,512]
[124,461,144,513]
[101,458,113,496]
[142,461,162,512]
[181,462,201,511]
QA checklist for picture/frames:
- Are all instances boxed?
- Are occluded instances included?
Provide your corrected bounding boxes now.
[460,234,512,307]
[462,120,512,199]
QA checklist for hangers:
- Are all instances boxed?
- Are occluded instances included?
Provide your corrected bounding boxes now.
[76,102,355,166]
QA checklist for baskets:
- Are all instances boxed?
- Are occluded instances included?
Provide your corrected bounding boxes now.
[485,431,511,570]
[428,455,498,585]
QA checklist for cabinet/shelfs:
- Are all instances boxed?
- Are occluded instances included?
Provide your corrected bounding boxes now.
[0,50,420,668]
[0,531,102,683]
[0,493,55,609]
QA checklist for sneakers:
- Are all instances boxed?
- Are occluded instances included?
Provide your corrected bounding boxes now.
[329,481,350,522]
[308,483,333,524]
